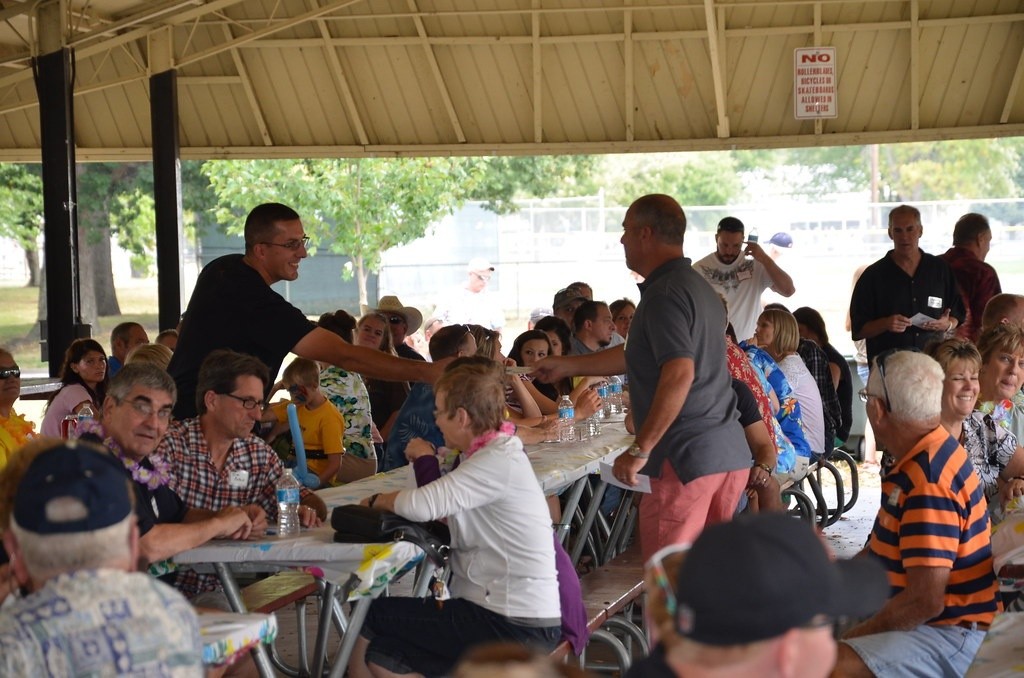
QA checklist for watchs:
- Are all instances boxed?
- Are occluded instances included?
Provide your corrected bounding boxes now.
[627,443,649,459]
[367,492,380,509]
[755,463,772,477]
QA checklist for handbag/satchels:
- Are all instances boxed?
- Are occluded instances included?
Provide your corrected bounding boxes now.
[330,501,451,569]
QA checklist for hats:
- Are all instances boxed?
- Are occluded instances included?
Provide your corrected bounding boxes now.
[672,515,888,642]
[13,441,133,534]
[553,289,591,311]
[362,296,423,336]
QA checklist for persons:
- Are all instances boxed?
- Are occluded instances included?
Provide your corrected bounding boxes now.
[850,205,967,370]
[531,194,756,648]
[73,361,268,593]
[0,441,206,678]
[927,339,1024,524]
[936,213,1002,341]
[620,510,887,678]
[167,204,460,440]
[347,356,577,678]
[0,281,855,537]
[829,349,1003,678]
[155,349,327,678]
[975,293,1024,449]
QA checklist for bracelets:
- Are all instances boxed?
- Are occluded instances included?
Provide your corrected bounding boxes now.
[946,321,953,332]
[1007,476,1024,482]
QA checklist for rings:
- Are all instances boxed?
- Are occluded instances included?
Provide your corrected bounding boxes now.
[762,478,767,483]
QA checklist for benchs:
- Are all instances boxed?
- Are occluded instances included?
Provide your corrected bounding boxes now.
[544,443,859,678]
[237,567,320,614]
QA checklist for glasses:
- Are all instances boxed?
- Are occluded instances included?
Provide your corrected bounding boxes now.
[876,347,926,413]
[259,237,310,251]
[456,324,471,349]
[115,397,174,426]
[385,316,406,325]
[858,388,888,415]
[216,392,271,411]
[0,369,21,379]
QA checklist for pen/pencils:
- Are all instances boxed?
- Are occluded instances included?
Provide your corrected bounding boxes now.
[265,527,306,532]
[266,531,276,536]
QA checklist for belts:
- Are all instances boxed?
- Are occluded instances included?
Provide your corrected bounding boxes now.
[960,621,990,630]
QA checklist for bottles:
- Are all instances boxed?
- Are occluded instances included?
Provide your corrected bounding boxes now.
[556,395,575,443]
[276,468,301,539]
[586,411,602,437]
[592,380,611,419]
[607,375,623,415]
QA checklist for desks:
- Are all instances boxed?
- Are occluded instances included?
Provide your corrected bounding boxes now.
[170,388,640,678]
